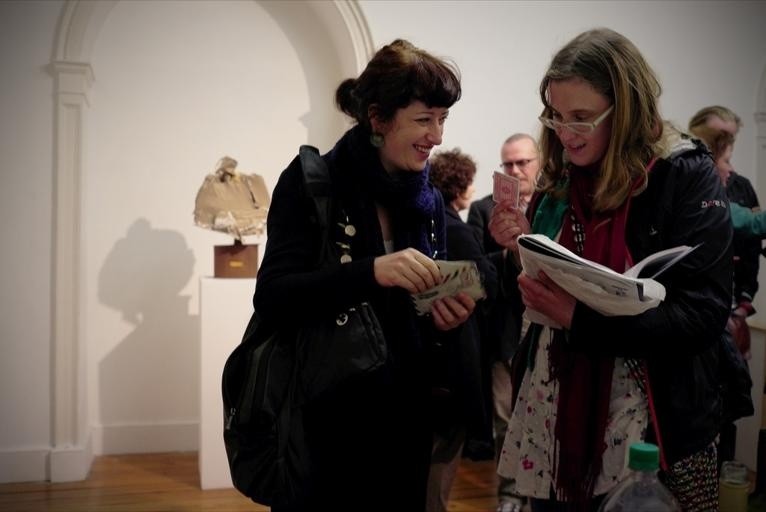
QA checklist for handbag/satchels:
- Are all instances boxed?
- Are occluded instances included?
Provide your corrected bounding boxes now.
[222,305,386,506]
[656,440,719,507]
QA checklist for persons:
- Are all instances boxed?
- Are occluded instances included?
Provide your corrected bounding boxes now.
[482,26,756,511]
[688,105,764,460]
[467,132,545,511]
[426,145,510,511]
[684,120,766,244]
[247,38,482,512]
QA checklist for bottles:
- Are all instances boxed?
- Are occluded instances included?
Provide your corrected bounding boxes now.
[718,461,752,512]
[595,444,681,512]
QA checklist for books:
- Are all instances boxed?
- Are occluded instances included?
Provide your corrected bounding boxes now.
[508,223,701,330]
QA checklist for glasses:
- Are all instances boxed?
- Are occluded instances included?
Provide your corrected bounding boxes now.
[499,158,536,170]
[538,107,613,134]
